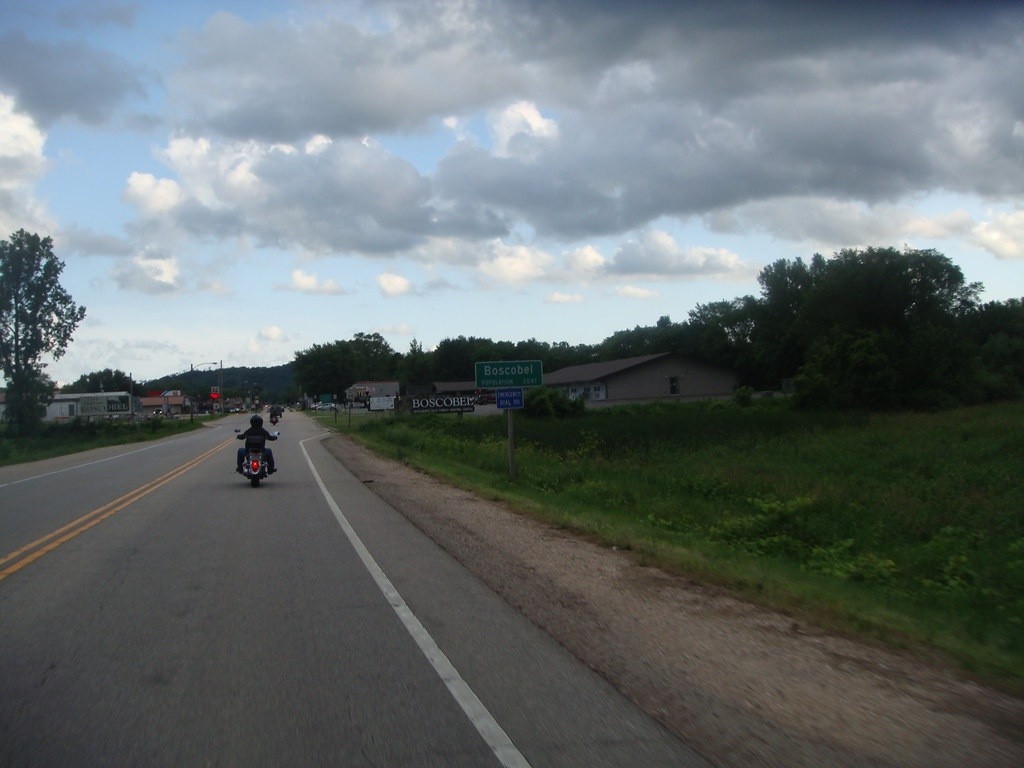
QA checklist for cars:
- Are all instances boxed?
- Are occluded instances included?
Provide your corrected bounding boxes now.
[475,390,496,405]
[153,409,162,414]
[311,402,322,410]
[270,406,282,418]
[317,403,335,411]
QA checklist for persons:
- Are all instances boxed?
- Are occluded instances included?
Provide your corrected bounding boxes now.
[236,415,278,476]
[269,410,280,423]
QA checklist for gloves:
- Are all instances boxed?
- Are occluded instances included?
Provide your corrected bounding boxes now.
[273,431,279,437]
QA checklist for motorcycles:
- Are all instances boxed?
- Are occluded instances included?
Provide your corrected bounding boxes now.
[270,414,279,425]
[234,428,280,488]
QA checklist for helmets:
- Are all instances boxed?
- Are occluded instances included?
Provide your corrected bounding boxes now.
[250,415,263,427]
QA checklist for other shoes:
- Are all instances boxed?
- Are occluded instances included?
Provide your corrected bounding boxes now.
[236,468,243,473]
[267,468,277,474]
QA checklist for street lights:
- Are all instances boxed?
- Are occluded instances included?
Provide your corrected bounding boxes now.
[191,362,217,424]
